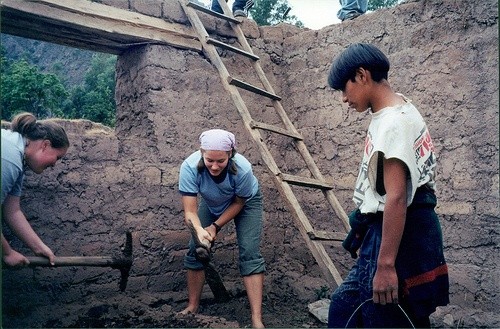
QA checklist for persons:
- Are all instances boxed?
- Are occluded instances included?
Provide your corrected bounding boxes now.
[211,0,255,16]
[177,128,263,328]
[0,112,69,269]
[327,42,449,329]
[337,0,369,22]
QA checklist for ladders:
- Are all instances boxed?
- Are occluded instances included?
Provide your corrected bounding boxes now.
[181,0,361,291]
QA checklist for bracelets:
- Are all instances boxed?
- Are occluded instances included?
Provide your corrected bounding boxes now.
[213,222,221,235]
